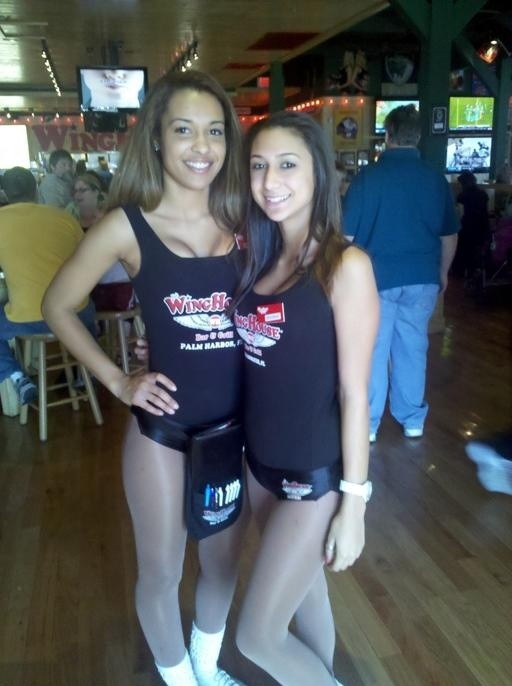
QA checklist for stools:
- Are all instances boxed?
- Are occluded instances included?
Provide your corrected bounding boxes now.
[11,304,151,445]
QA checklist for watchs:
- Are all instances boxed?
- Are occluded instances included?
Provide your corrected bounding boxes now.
[339,478,374,504]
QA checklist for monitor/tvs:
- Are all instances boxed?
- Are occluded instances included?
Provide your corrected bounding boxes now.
[77,66,147,114]
[372,138,386,164]
[448,95,495,134]
[444,135,494,173]
[373,96,424,136]
[83,113,128,132]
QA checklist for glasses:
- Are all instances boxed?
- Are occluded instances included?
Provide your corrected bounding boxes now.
[72,187,94,194]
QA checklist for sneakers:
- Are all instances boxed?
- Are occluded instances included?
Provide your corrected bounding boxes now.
[12,374,38,407]
[198,668,239,686]
[72,375,96,391]
[403,425,424,438]
[465,440,512,499]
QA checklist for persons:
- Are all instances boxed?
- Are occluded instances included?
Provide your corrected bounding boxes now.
[40,68,250,686]
[82,71,146,112]
[341,104,464,444]
[455,169,491,274]
[0,149,135,407]
[135,112,379,686]
[480,192,511,278]
